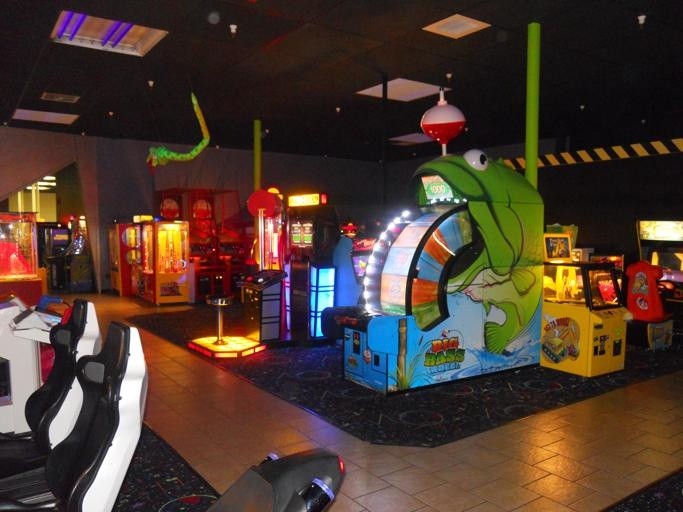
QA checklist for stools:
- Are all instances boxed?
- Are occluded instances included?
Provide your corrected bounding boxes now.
[205,293,233,347]
